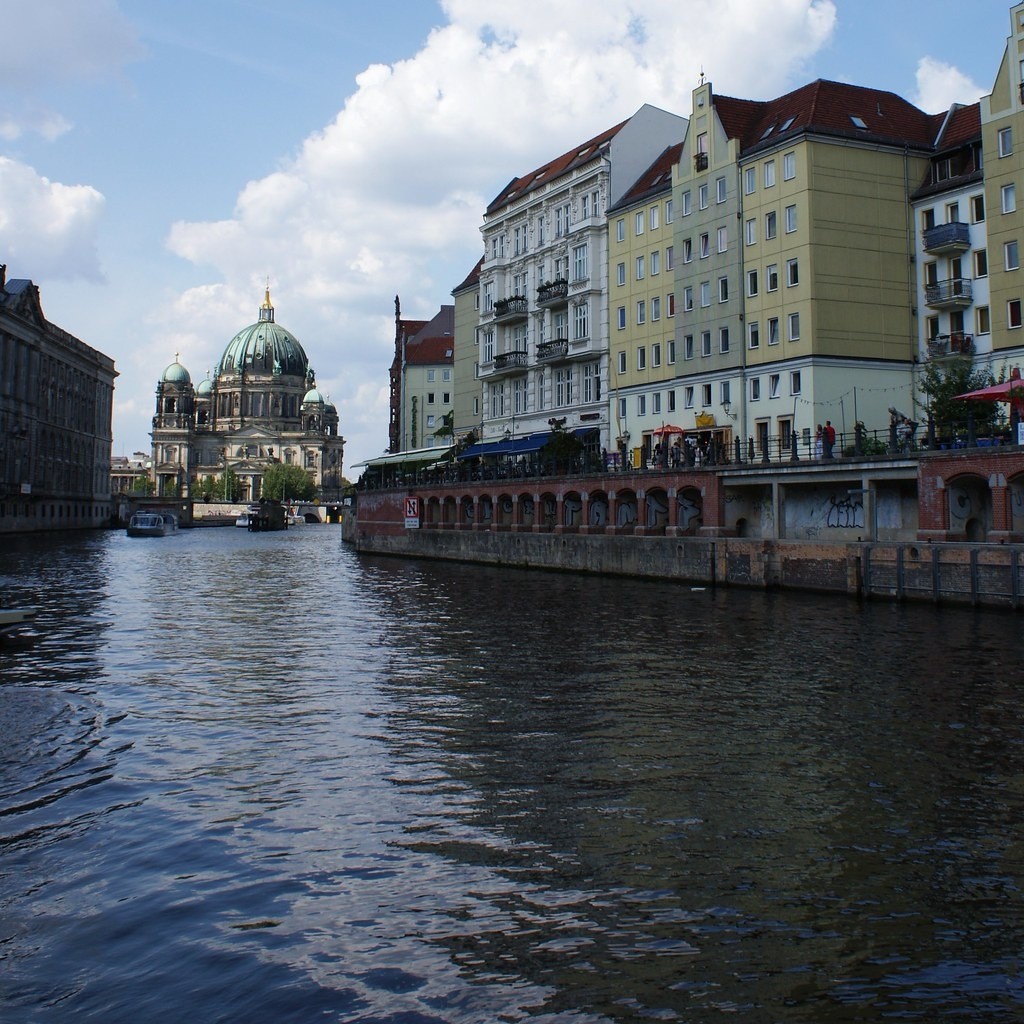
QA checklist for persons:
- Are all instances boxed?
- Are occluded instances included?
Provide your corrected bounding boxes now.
[812,419,914,458]
[359,439,718,490]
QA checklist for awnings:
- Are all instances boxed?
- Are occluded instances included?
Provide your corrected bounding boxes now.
[353,426,597,470]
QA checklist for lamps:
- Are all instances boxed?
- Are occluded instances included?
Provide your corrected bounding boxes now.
[504,428,515,439]
[721,399,737,420]
[623,430,630,439]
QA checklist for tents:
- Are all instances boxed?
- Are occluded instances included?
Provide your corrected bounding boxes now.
[954,380,1024,402]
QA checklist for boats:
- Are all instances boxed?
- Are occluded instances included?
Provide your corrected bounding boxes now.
[235,511,251,527]
[0,603,44,623]
[127,509,179,536]
